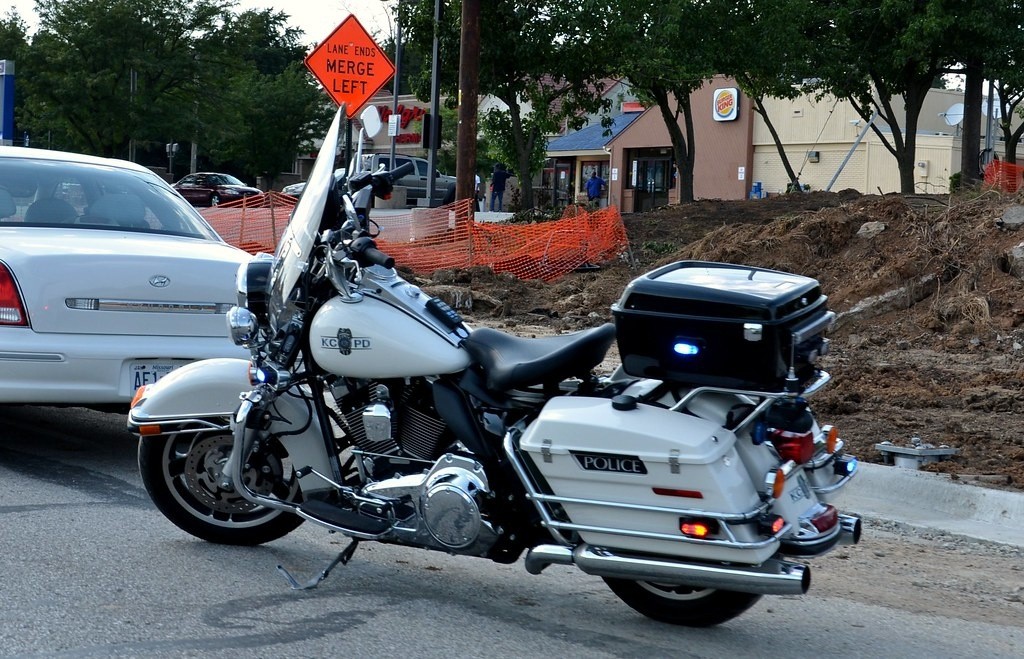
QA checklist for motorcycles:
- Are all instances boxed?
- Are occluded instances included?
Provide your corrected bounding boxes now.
[126,102,865,628]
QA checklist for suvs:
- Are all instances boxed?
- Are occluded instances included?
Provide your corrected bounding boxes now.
[352,153,456,207]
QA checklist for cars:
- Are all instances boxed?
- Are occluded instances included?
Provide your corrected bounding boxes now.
[280,167,345,200]
[169,172,266,210]
[1,141,260,417]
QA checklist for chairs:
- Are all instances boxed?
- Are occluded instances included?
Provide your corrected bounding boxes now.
[24,198,79,224]
[89,191,147,228]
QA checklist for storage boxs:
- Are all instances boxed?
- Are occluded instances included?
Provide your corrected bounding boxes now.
[614,262,835,392]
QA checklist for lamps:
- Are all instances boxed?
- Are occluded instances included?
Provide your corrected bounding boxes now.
[809,151,820,163]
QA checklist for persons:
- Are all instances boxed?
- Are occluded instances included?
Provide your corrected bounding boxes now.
[584,171,607,210]
[490,162,511,212]
